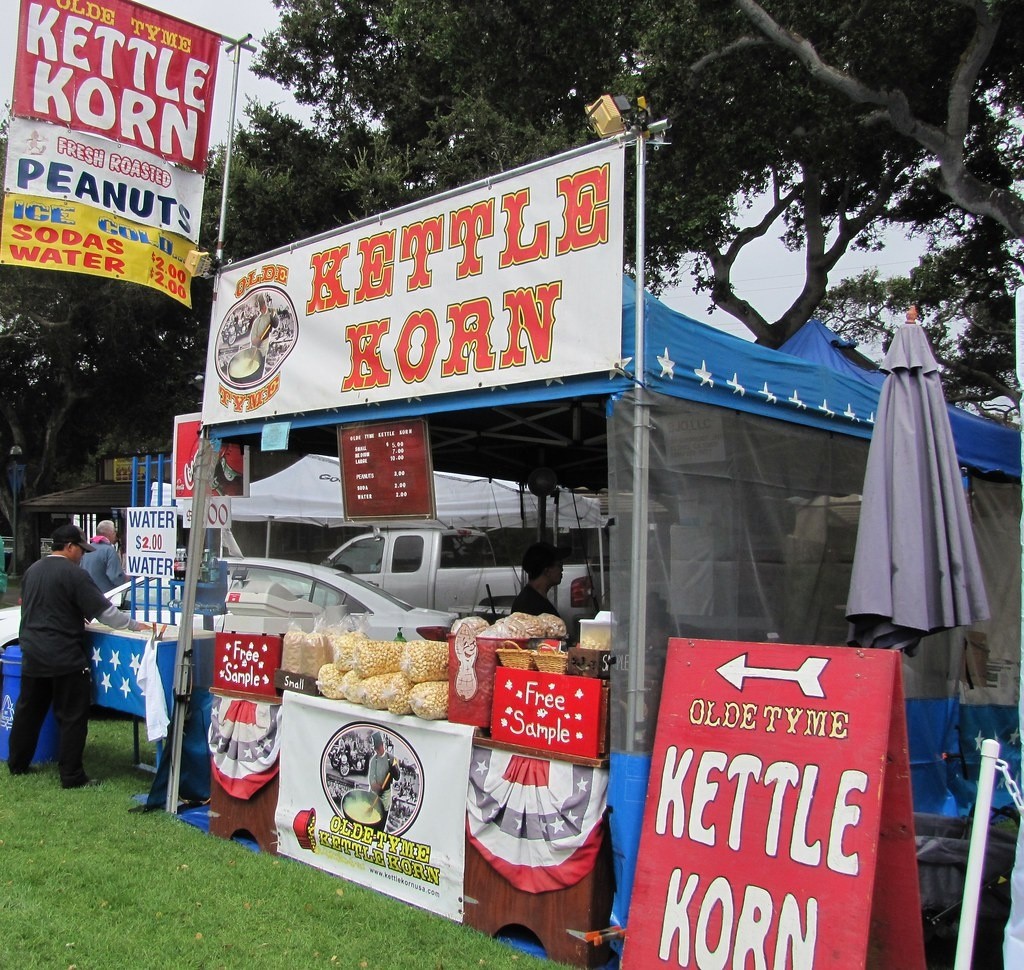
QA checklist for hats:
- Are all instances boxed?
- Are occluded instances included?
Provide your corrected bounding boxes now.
[522,543,572,578]
[52,524,96,553]
[371,731,385,749]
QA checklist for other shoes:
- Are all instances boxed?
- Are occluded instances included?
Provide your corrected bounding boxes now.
[7,766,41,776]
[61,778,100,788]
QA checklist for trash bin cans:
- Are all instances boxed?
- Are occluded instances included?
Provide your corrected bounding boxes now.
[0,645,59,762]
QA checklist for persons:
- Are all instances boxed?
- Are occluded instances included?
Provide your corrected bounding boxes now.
[79,520,130,595]
[512,543,564,616]
[8,524,152,789]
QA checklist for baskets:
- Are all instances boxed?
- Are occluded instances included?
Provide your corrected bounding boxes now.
[493,640,533,671]
[530,642,569,676]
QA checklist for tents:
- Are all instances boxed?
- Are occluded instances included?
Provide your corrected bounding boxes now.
[136,259,1023,952]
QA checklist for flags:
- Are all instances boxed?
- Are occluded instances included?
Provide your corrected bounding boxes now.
[222,526,244,559]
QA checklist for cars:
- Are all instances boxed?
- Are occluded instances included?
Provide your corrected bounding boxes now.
[1,557,456,656]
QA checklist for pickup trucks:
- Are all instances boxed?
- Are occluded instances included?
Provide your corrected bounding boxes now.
[292,526,596,640]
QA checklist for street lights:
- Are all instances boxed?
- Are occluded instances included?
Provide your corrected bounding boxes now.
[7,445,24,581]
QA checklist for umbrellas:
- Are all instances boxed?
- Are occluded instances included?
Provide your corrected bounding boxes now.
[842,311,992,657]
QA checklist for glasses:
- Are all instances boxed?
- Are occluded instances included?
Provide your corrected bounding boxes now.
[555,562,564,568]
